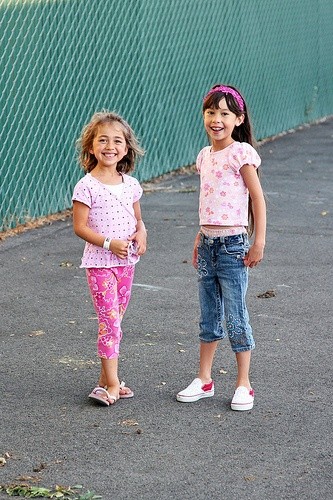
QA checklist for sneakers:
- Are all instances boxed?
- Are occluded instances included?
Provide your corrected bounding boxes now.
[231,385,256,411]
[176,377,216,405]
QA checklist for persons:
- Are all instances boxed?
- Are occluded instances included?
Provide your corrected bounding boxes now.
[70,112,146,406]
[176,85,266,410]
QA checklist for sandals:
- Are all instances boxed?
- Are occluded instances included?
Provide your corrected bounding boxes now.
[94,381,135,399]
[86,387,115,406]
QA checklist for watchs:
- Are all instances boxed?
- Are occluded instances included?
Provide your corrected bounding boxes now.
[103,236,112,251]
